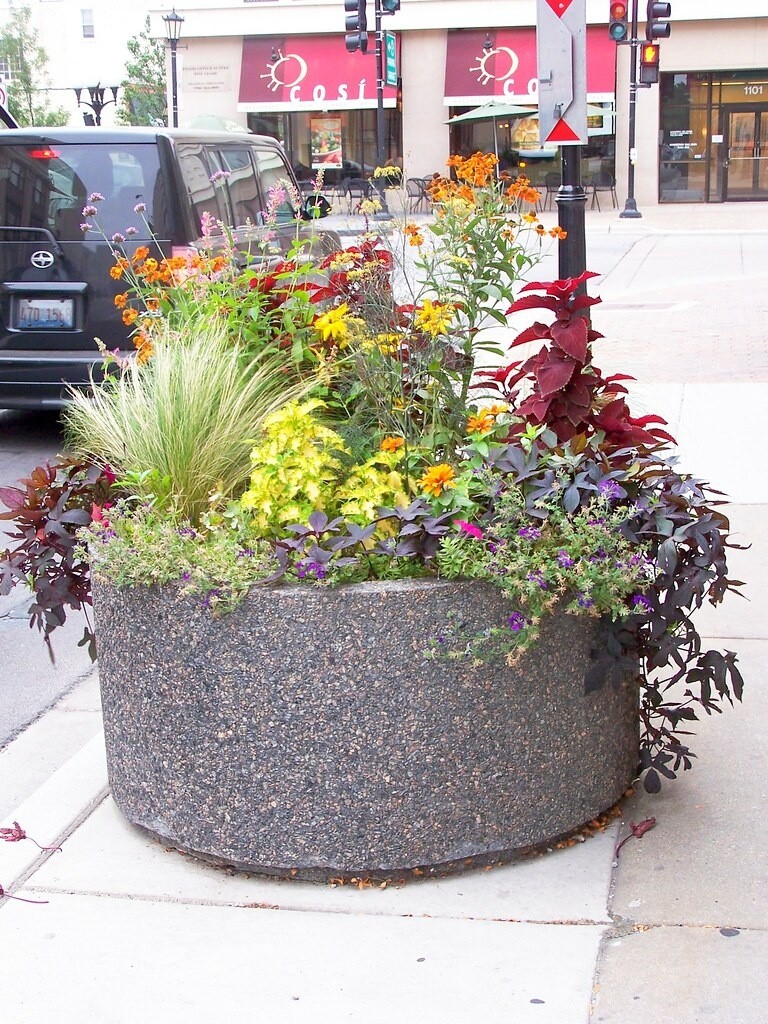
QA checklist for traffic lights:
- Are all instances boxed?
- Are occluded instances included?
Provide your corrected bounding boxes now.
[639,43,661,83]
[380,0,401,12]
[342,0,369,54]
[609,0,630,41]
[645,0,672,42]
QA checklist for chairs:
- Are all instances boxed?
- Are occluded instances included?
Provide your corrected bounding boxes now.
[405,172,619,216]
[300,176,378,217]
[55,155,114,241]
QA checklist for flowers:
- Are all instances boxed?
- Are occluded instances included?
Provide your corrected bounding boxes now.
[0,150,748,791]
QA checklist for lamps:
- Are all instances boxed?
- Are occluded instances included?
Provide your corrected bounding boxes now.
[483,33,493,49]
[270,41,284,62]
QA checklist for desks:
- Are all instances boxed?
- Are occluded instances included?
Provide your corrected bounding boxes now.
[297,180,310,194]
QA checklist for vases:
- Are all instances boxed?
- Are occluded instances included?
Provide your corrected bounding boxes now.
[82,528,644,878]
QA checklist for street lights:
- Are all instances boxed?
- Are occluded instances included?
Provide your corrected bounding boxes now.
[161,6,184,129]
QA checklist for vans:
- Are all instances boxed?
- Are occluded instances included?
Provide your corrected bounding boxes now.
[0,127,332,420]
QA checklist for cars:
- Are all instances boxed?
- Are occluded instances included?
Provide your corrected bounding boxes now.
[294,158,379,185]
